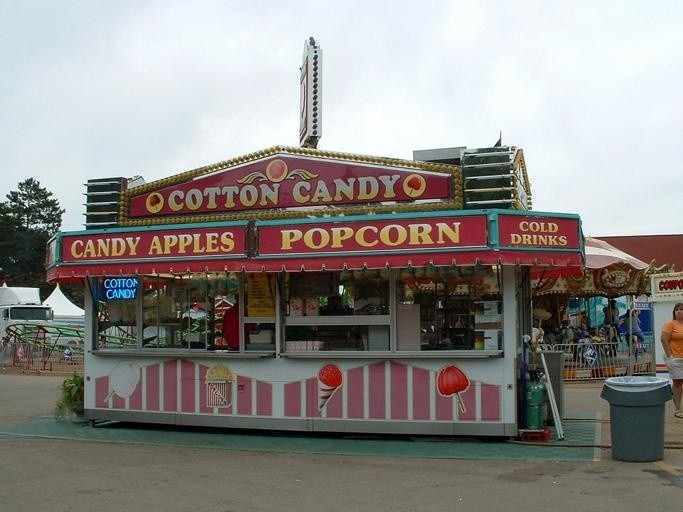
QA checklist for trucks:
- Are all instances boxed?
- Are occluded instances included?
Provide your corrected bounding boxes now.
[0,286,54,346]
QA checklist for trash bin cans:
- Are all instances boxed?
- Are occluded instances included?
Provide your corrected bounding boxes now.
[600,376,674,462]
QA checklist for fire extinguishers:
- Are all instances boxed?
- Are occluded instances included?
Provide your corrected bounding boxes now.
[524,369,547,430]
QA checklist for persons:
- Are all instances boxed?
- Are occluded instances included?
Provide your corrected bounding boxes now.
[65,348,73,364]
[603,300,623,342]
[625,310,644,356]
[17,345,24,361]
[661,303,683,419]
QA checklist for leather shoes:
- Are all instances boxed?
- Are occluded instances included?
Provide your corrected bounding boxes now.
[674,409,683,418]
